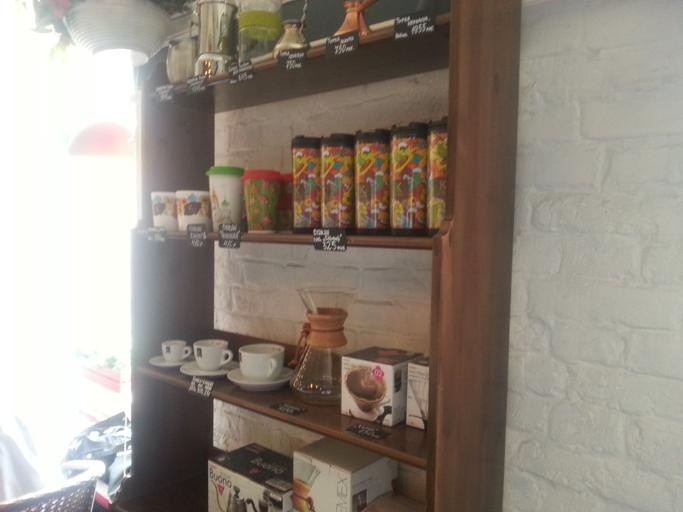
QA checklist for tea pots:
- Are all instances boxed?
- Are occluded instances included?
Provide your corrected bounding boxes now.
[211,480,259,511]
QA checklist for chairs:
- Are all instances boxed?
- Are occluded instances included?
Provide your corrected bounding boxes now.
[0,457,105,511]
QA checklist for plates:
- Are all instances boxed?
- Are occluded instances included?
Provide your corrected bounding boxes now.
[227,367,294,392]
[179,360,239,378]
[148,355,187,369]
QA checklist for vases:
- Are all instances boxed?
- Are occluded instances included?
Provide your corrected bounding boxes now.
[61,2,170,67]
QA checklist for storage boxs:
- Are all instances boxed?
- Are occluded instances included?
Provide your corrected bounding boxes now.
[206,437,428,512]
[340,344,429,431]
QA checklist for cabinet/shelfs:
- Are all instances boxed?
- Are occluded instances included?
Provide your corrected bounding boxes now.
[124,1,521,512]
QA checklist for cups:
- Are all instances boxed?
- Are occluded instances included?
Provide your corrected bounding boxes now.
[238,343,285,380]
[204,166,244,233]
[191,339,234,371]
[175,191,211,233]
[150,191,177,229]
[346,367,388,412]
[160,339,192,364]
[241,170,283,234]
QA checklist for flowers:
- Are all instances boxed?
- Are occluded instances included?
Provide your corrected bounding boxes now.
[32,1,192,56]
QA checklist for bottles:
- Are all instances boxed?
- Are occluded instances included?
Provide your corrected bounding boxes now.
[288,284,358,408]
[353,128,390,236]
[389,123,427,236]
[427,117,447,237]
[237,9,282,63]
[318,132,353,236]
[289,135,320,235]
